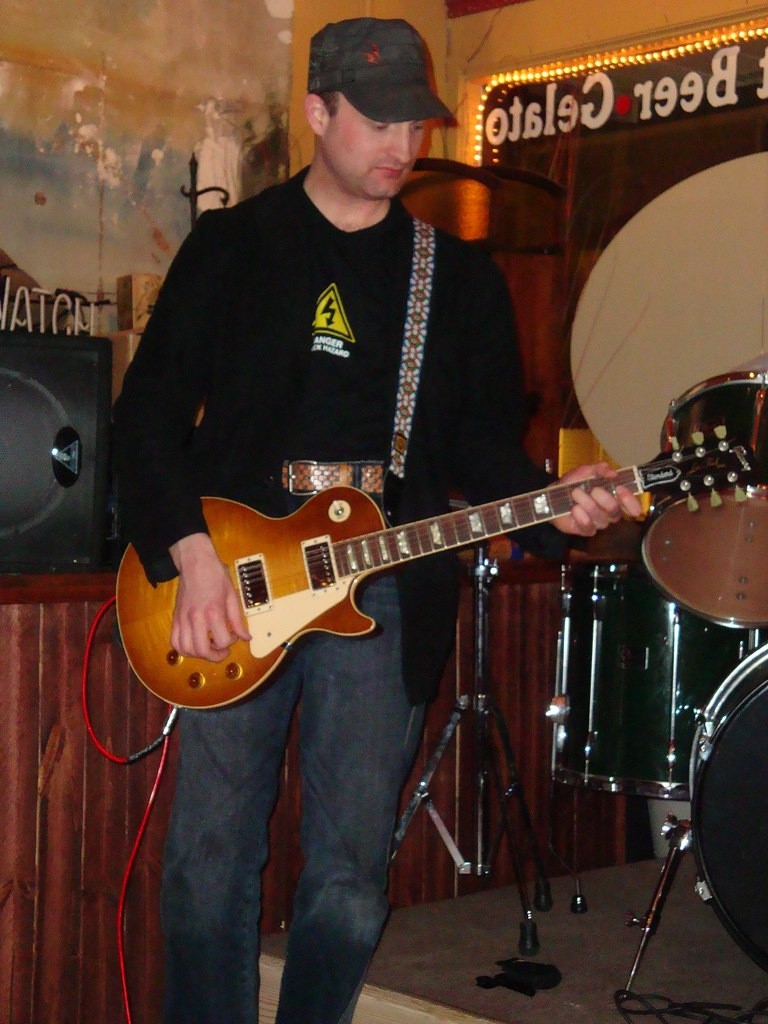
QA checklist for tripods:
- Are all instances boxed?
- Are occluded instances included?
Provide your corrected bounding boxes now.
[388,540,554,958]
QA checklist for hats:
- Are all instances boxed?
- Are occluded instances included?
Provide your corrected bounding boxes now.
[307,17,458,126]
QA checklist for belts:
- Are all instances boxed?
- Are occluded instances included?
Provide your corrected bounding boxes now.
[252,460,389,498]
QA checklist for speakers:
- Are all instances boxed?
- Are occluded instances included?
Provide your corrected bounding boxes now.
[0,329,114,572]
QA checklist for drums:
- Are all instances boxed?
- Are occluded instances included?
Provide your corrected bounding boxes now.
[552,558,748,799]
[637,371,768,630]
[686,643,768,972]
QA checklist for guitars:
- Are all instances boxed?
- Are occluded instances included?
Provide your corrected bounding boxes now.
[115,424,757,711]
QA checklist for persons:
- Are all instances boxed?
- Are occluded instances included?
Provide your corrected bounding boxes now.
[116,18,642,1024]
